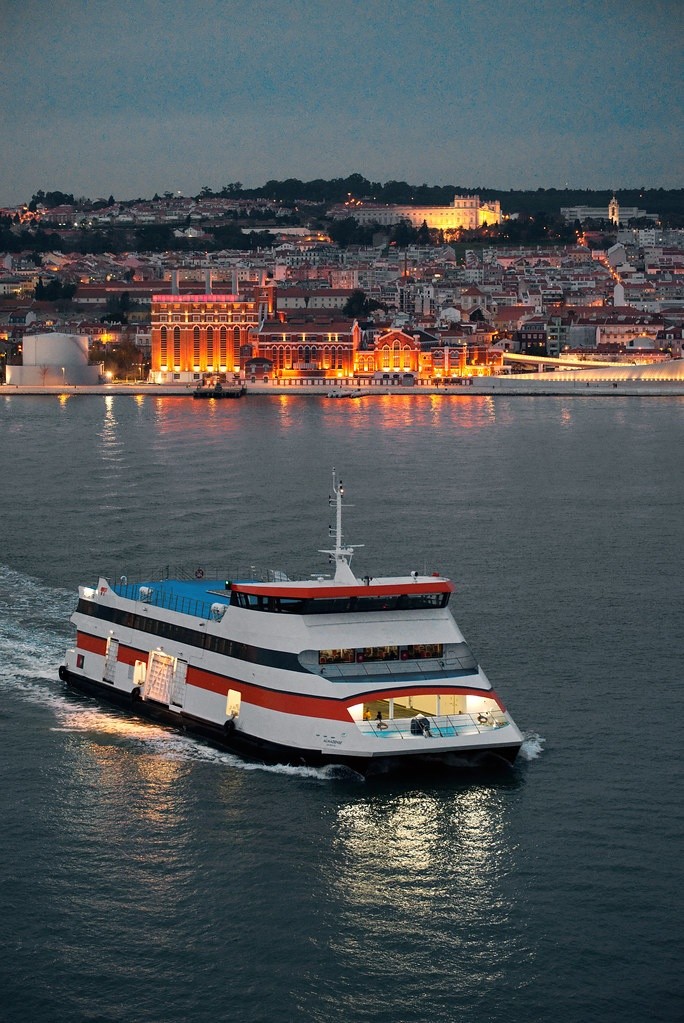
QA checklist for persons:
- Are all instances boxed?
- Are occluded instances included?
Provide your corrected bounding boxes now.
[363,706,372,720]
[375,711,382,719]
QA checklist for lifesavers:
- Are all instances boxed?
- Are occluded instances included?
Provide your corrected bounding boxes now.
[195,568,204,578]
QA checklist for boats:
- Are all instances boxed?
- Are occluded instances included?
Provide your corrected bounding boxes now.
[57,465,526,783]
